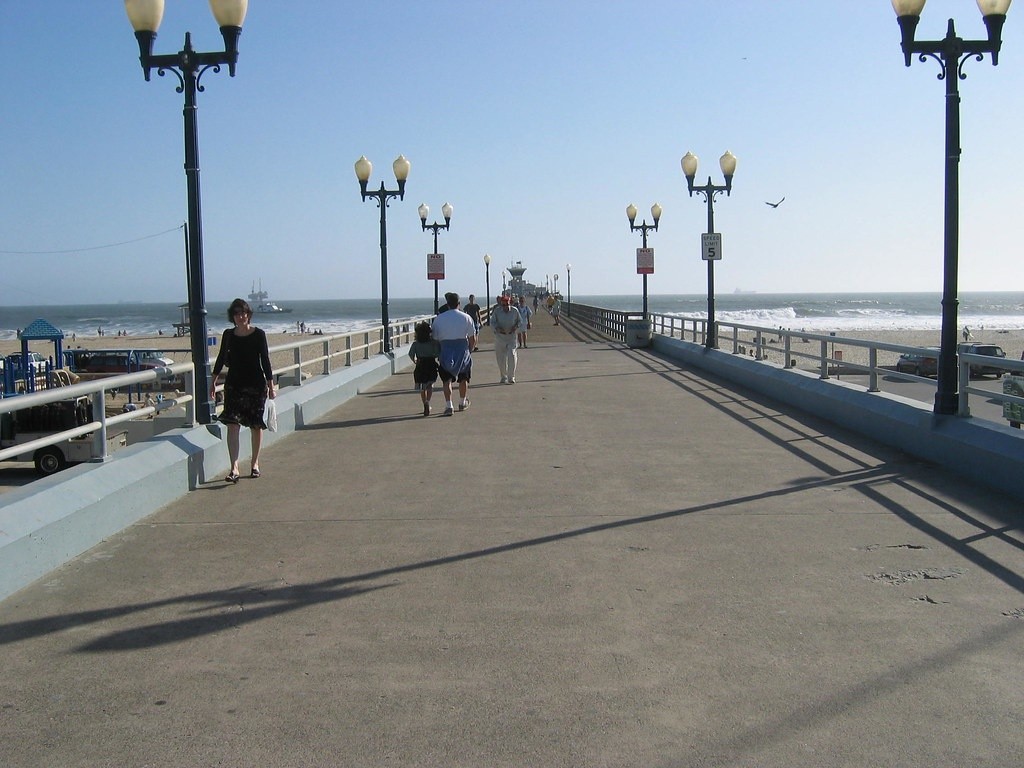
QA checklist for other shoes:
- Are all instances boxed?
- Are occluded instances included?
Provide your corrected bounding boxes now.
[500,378,508,384]
[444,407,454,416]
[474,347,478,351]
[458,399,471,411]
[508,376,515,383]
[553,322,560,325]
[423,402,432,415]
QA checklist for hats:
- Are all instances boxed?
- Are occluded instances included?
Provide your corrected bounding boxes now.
[501,296,510,302]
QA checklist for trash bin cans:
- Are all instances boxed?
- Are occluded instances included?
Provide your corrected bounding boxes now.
[207,337,217,345]
[625,319,652,349]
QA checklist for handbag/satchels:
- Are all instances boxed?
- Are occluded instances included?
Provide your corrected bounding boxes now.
[527,322,532,330]
[263,397,278,432]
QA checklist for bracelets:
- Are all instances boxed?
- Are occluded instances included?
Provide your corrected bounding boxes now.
[270,387,275,391]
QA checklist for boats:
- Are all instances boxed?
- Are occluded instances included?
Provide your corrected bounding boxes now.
[246,277,294,314]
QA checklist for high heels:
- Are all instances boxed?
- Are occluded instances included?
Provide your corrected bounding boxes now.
[251,468,261,478]
[226,471,239,484]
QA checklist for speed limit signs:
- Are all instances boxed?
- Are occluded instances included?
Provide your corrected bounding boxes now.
[701,232,722,261]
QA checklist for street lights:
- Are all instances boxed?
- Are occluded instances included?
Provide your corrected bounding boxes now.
[892,0,1012,415]
[566,264,572,317]
[355,153,411,352]
[126,0,250,424]
[418,202,453,322]
[484,254,491,325]
[502,271,507,297]
[680,149,743,344]
[626,202,663,318]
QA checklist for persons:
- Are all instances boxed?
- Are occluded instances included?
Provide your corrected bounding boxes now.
[72,333,76,341]
[17,328,21,337]
[432,293,476,415]
[533,296,538,314]
[118,329,127,336]
[159,329,162,335]
[749,350,754,357]
[301,322,323,335]
[98,326,104,336]
[491,294,532,383]
[409,322,438,416]
[143,393,155,419]
[963,326,969,341]
[463,295,483,351]
[123,404,137,413]
[210,298,276,483]
[778,326,805,342]
[547,296,561,325]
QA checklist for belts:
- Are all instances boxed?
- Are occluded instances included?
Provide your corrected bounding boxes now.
[501,332,512,334]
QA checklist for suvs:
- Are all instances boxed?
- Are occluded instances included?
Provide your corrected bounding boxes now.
[6,350,51,377]
[896,345,940,378]
[956,341,1007,378]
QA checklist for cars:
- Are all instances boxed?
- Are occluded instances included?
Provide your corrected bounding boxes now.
[86,350,176,373]
